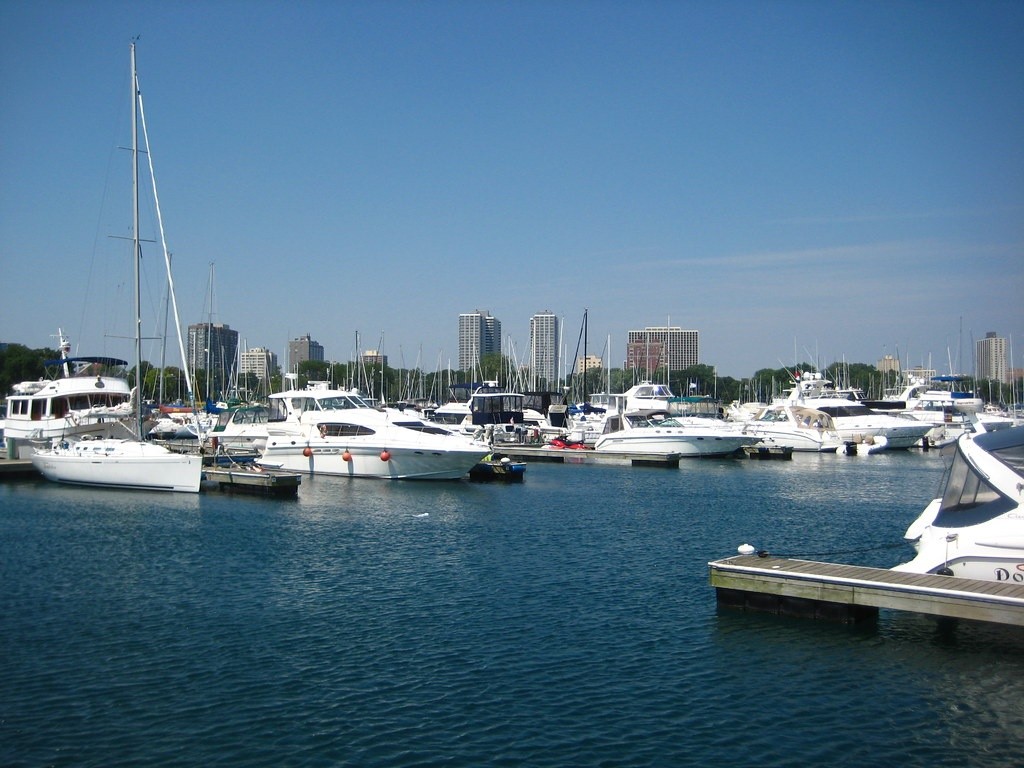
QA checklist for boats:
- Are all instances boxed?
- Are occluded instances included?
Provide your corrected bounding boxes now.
[888,423,1024,588]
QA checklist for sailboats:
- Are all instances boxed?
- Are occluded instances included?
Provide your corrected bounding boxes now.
[0,33,1023,494]
